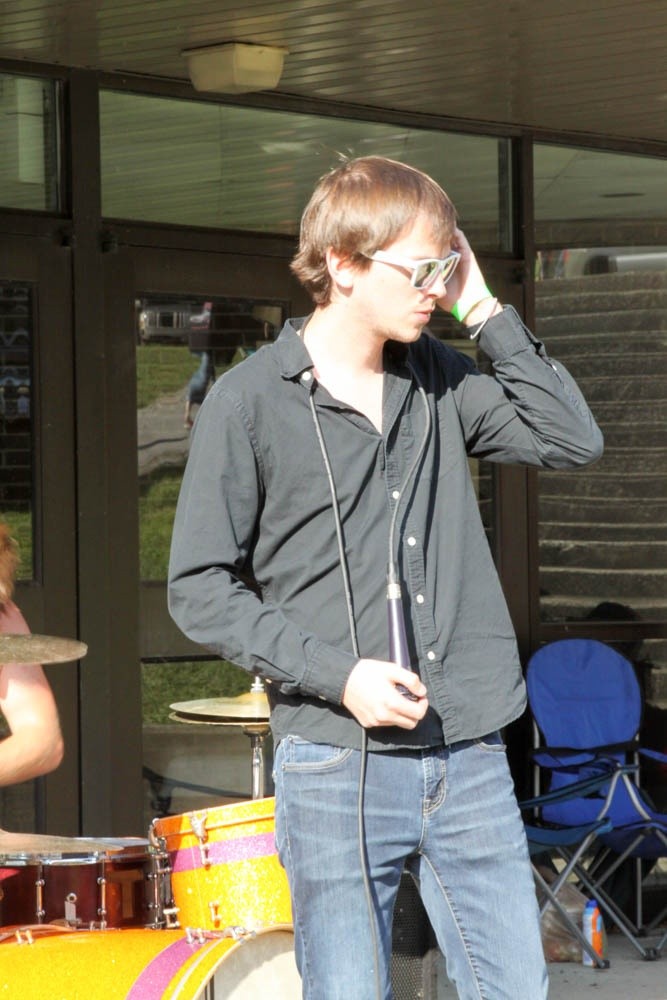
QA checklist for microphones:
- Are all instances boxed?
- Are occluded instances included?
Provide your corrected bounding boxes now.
[385,575,419,703]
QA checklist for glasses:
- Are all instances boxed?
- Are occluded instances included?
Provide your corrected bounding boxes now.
[359,251,461,291]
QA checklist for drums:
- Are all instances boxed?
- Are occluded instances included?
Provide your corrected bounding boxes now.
[0,924,304,1000]
[155,796,294,931]
[0,833,171,928]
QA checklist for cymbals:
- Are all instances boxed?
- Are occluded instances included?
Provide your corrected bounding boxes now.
[168,692,271,739]
[0,632,89,665]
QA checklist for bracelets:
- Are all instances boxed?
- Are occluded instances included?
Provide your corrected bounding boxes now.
[450,284,492,322]
[470,297,498,339]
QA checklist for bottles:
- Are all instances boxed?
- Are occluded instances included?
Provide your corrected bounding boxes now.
[582,900,608,967]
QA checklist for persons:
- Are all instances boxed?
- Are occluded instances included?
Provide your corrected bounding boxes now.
[168,151,607,1000]
[0,520,63,787]
[182,300,216,430]
[507,603,667,933]
